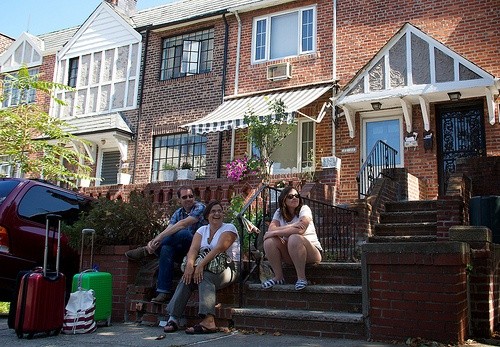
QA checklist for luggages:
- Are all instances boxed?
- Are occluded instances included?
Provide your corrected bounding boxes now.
[71,229,113,326]
[7,214,67,339]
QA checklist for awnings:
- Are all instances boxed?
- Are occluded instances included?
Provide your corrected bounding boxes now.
[178,83,335,135]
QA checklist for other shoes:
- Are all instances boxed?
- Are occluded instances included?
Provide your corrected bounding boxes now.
[262,278,285,289]
[295,280,307,290]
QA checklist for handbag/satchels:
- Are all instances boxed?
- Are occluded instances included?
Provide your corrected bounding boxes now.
[181,247,232,274]
[60,289,98,334]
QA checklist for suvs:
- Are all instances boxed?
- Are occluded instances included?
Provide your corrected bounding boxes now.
[0,176,94,305]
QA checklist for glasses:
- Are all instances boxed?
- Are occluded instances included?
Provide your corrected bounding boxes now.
[209,210,222,213]
[180,194,194,200]
[286,194,299,199]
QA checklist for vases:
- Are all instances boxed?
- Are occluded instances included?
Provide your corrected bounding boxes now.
[321,157,342,169]
[77,178,91,187]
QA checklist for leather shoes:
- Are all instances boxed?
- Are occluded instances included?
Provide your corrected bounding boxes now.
[151,292,172,304]
[124,247,154,262]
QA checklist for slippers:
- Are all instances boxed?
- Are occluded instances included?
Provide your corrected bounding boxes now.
[164,320,177,332]
[185,323,217,334]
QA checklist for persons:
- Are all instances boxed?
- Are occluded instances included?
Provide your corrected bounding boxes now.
[164,200,241,334]
[133,185,210,304]
[261,185,324,291]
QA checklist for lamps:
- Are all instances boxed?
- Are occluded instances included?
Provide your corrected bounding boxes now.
[102,139,106,145]
[447,91,463,103]
[371,102,382,111]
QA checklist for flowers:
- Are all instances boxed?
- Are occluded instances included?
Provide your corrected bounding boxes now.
[226,153,268,182]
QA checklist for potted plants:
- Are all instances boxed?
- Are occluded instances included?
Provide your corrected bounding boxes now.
[159,163,175,181]
[116,160,131,185]
[177,161,196,180]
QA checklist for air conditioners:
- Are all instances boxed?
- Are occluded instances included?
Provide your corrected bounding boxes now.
[267,63,294,83]
